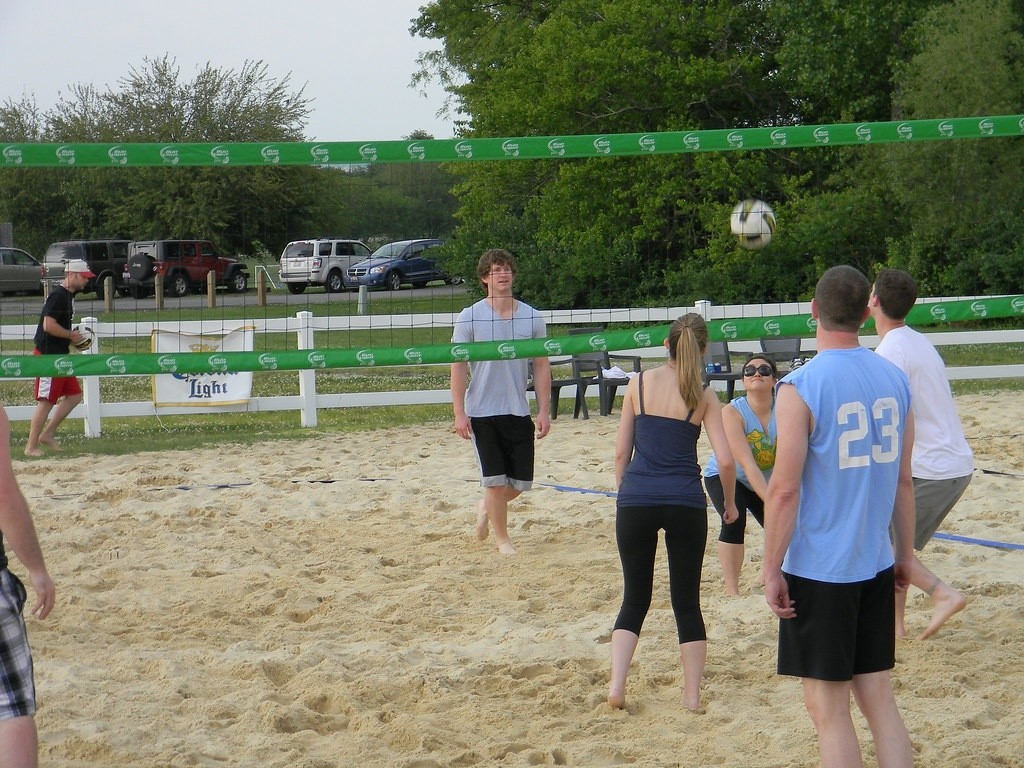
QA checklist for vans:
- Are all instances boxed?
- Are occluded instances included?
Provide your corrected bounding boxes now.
[278,238,374,294]
[42,240,136,301]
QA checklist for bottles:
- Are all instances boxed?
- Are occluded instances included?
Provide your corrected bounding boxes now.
[707,364,714,373]
[714,361,721,373]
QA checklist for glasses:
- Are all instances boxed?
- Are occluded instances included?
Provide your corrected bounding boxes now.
[744,365,775,377]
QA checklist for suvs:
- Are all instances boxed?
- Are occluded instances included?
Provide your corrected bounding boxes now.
[122,240,250,298]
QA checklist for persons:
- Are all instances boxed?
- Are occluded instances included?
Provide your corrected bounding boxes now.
[705,355,784,594]
[25,259,98,459]
[606,313,737,707]
[868,270,974,640]
[763,265,915,768]
[0,405,55,768]
[451,250,551,554]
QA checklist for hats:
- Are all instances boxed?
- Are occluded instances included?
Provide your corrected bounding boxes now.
[64,259,97,278]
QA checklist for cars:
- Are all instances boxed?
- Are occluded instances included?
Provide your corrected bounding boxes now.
[344,238,464,291]
[0,246,44,294]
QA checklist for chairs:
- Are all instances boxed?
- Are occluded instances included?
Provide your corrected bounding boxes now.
[525,357,590,420]
[701,339,743,404]
[568,327,642,419]
[760,338,818,396]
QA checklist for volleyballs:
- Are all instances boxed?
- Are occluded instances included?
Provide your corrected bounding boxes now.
[72,324,96,351]
[730,197,776,249]
